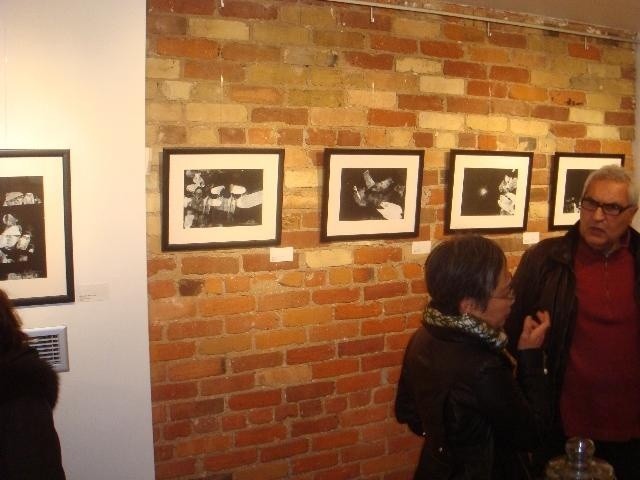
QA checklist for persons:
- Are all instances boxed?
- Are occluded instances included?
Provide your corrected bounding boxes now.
[397,234,555,480]
[362,185,383,220]
[500,167,639,479]
[0,289,66,480]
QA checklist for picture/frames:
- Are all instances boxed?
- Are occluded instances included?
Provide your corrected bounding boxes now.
[1,149,77,306]
[162,147,285,252]
[319,146,425,242]
[444,148,534,235]
[549,151,625,230]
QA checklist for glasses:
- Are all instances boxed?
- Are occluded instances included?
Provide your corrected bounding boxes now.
[480,273,517,303]
[580,193,633,216]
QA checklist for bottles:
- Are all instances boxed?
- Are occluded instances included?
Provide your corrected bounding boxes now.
[545,437,619,480]
